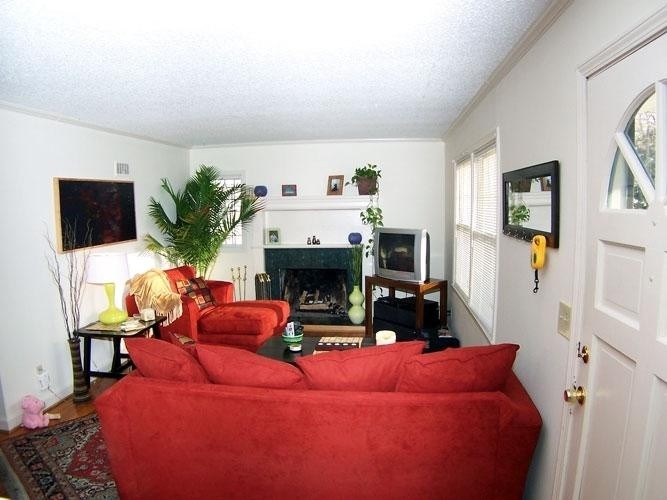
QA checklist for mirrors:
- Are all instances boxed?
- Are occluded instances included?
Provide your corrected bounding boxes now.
[502,160,560,249]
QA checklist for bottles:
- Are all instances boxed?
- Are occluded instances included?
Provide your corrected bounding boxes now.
[307,235,321,244]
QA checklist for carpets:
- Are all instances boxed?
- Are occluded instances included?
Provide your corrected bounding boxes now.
[0,409,121,500]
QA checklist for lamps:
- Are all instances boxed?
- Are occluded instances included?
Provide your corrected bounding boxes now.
[86,253,127,325]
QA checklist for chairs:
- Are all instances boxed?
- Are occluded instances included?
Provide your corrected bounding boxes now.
[124,265,291,350]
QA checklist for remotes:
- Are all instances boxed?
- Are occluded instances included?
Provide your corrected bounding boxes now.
[293,321,301,331]
[287,320,294,336]
[295,326,304,336]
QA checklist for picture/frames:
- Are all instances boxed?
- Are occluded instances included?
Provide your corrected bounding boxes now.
[265,227,281,246]
[326,175,344,196]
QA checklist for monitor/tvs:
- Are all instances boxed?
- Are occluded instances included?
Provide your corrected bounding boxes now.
[374,227,430,283]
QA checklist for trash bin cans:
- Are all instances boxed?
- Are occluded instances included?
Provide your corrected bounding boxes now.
[427,337,462,352]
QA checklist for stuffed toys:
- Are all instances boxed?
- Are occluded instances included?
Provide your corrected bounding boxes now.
[18,393,50,431]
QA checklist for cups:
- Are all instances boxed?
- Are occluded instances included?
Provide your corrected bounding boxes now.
[282,346,303,362]
[280,332,304,348]
[375,330,397,345]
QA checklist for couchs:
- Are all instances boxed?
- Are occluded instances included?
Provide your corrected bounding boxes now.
[87,364,546,500]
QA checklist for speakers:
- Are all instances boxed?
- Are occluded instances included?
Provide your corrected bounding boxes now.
[373,296,439,327]
[373,317,440,341]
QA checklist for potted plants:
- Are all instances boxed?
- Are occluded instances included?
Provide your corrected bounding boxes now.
[343,162,387,261]
[341,242,369,326]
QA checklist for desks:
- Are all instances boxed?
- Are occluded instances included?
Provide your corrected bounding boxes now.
[360,271,448,338]
[72,315,170,383]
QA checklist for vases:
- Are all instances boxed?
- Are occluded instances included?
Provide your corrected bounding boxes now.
[66,338,94,404]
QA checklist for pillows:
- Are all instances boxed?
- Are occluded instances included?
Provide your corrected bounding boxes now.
[172,272,218,311]
[291,334,426,393]
[189,335,310,389]
[120,331,213,387]
[389,336,521,397]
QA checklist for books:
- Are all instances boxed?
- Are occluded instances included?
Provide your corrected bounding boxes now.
[120,319,145,333]
